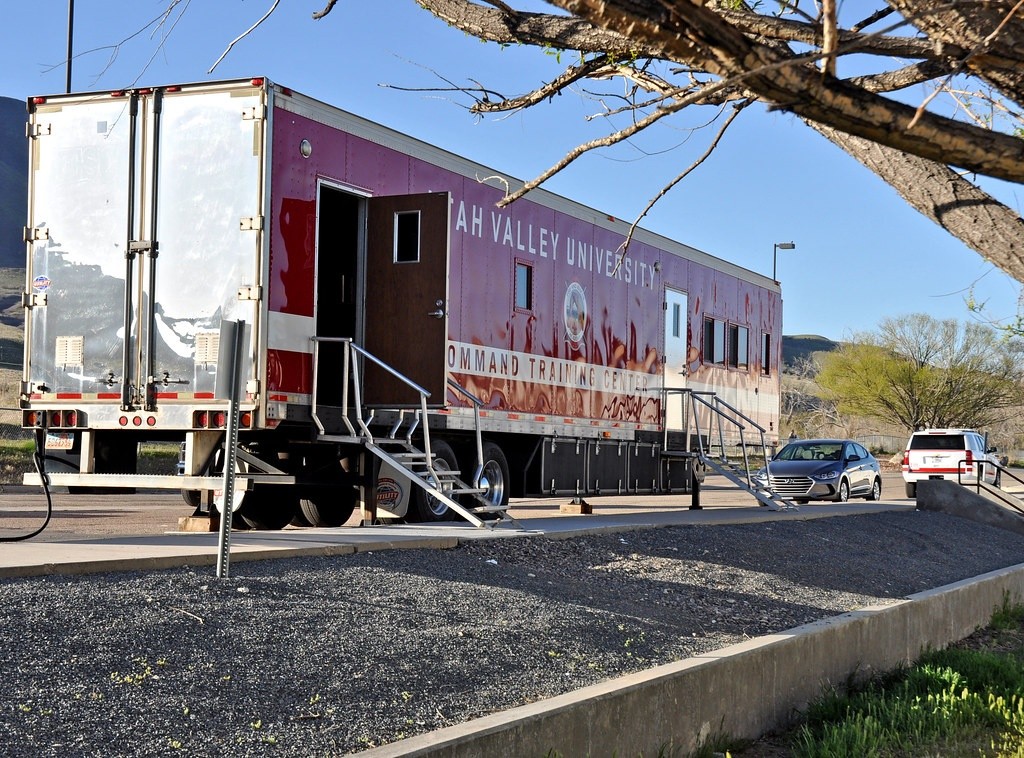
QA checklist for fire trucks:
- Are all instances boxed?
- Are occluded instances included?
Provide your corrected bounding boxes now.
[15,76,800,580]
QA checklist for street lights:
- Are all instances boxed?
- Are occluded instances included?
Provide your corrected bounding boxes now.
[773,242,797,280]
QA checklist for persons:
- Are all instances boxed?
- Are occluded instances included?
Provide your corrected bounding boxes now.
[788,429,798,445]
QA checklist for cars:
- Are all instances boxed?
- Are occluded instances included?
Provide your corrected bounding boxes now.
[756,439,883,507]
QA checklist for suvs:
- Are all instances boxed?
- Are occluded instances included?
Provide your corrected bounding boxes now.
[901,429,1010,497]
